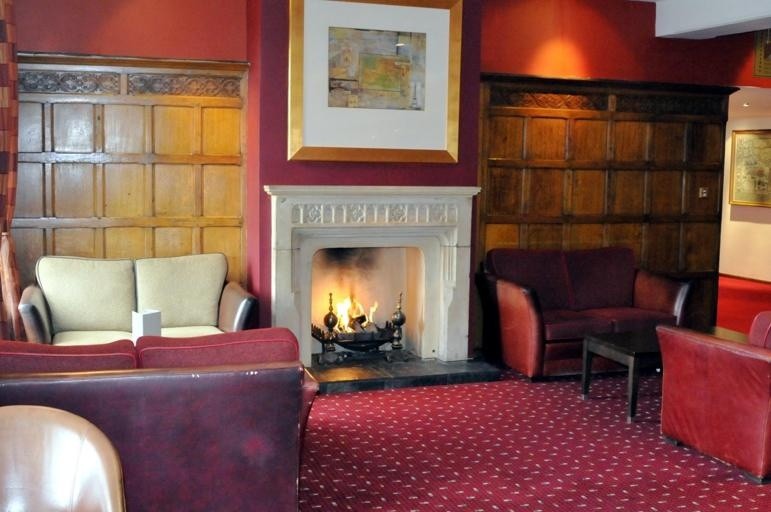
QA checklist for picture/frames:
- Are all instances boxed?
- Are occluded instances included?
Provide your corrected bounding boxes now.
[730,128,771,208]
[288,0,464,167]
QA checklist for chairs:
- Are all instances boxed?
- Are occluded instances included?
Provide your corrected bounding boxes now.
[655,308,771,483]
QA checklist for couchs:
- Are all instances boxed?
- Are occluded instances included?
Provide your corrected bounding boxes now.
[476,238,692,383]
[0,253,320,511]
